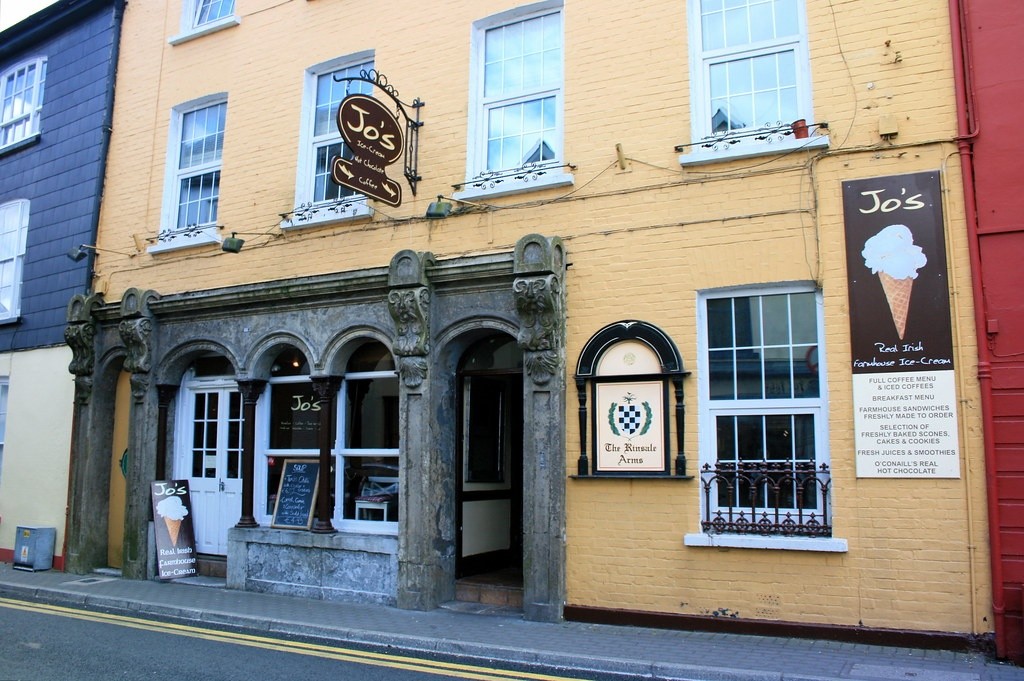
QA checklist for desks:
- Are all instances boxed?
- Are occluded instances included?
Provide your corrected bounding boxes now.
[355,500,395,521]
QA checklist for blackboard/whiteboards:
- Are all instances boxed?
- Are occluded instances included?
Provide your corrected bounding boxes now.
[269,459,320,531]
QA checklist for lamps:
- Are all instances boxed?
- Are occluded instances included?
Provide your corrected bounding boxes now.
[221,231,277,253]
[425,194,480,220]
[66,243,133,264]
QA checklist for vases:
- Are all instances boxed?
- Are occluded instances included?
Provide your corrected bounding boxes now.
[791,119,808,139]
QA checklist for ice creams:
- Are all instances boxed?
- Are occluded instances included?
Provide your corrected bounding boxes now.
[861,224,927,340]
[156,496,188,546]
